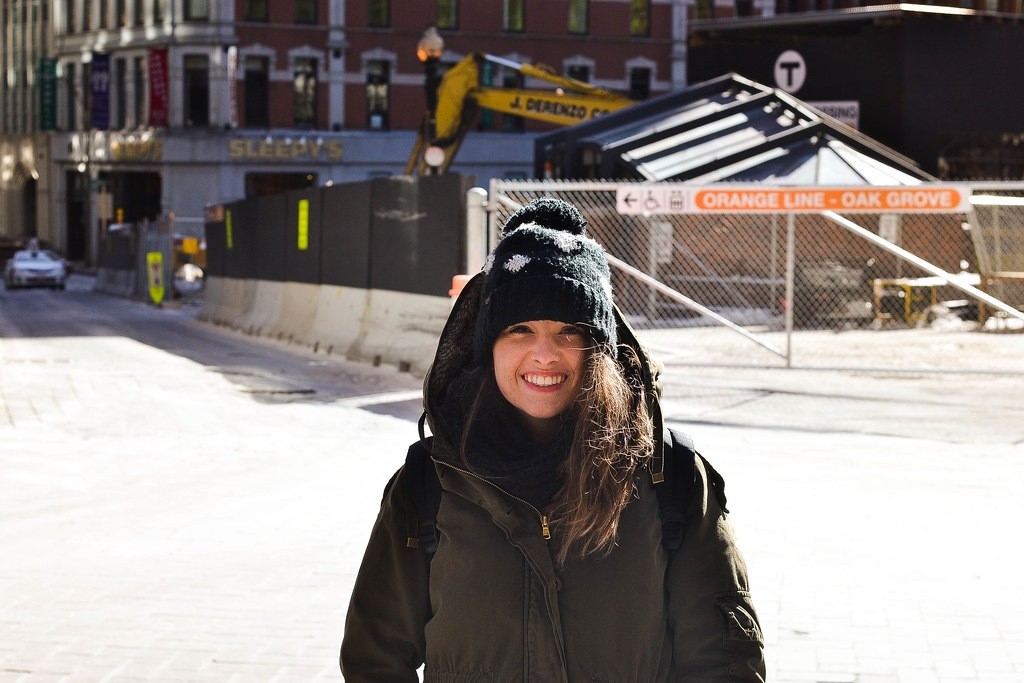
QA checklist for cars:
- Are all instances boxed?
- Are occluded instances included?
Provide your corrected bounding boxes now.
[4,250,67,291]
[780,257,906,328]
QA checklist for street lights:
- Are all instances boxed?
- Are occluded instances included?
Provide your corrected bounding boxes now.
[417,31,441,187]
[54,55,88,266]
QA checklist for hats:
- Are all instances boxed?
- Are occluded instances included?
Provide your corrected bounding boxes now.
[473,198,618,391]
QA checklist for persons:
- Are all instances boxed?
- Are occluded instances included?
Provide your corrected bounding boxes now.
[338,197,766,683]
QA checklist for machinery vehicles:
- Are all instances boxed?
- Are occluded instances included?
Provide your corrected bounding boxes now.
[400,55,642,185]
